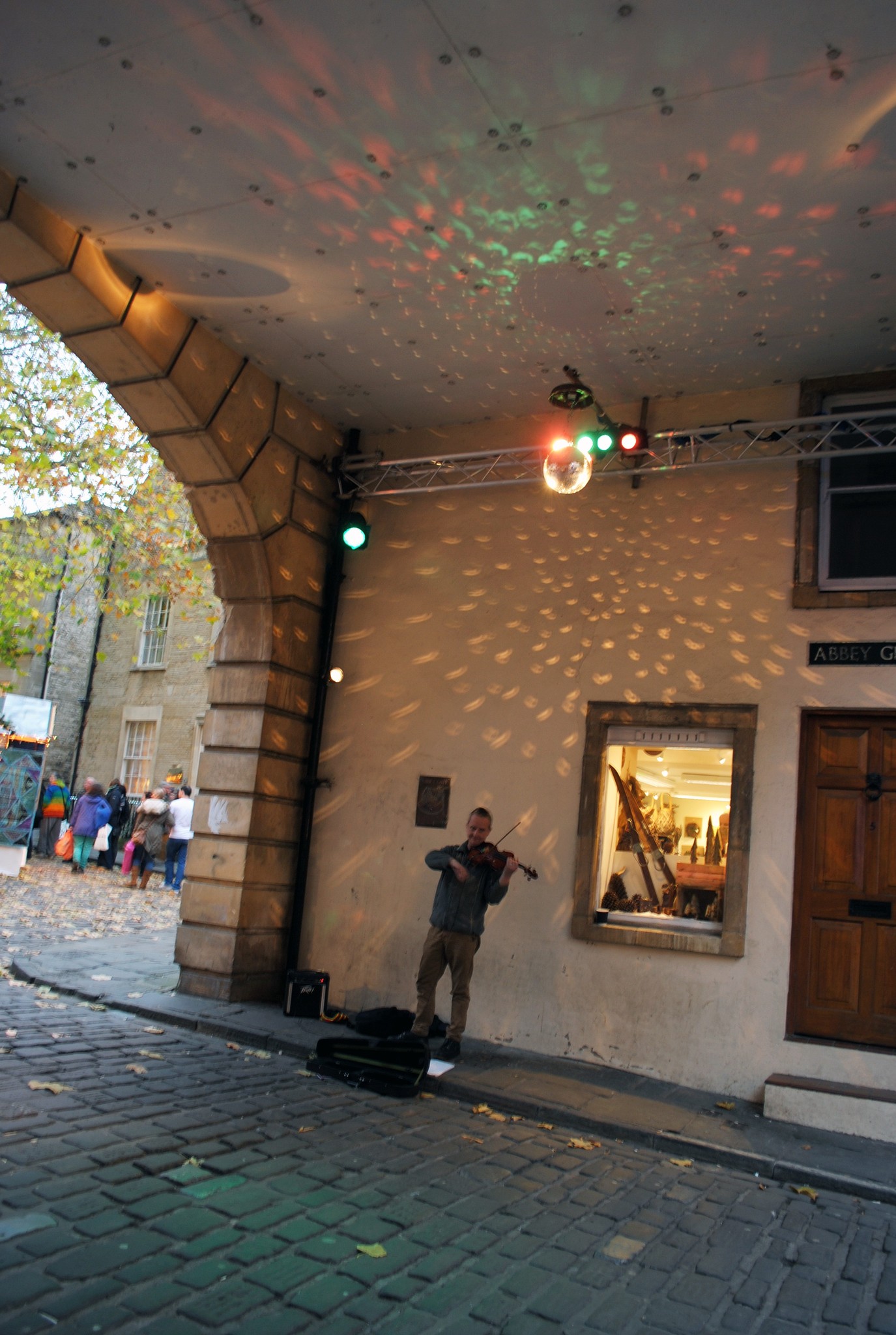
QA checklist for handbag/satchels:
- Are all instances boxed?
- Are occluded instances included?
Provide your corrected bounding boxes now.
[132,830,146,844]
[121,839,136,875]
[58,819,70,840]
[62,809,69,821]
[94,823,113,851]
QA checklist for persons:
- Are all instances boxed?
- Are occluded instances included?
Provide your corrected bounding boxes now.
[62,777,97,864]
[96,779,124,871]
[36,773,71,861]
[388,807,518,1057]
[158,785,195,894]
[68,784,112,875]
[95,785,179,879]
[124,786,175,890]
[14,775,46,858]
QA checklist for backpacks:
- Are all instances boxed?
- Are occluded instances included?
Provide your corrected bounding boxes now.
[93,798,111,831]
[107,789,130,826]
[55,828,73,860]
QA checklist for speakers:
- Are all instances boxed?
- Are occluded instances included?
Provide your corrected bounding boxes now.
[282,970,330,1018]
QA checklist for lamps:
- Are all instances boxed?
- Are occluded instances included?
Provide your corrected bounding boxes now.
[343,510,370,552]
[319,665,344,686]
[542,382,640,494]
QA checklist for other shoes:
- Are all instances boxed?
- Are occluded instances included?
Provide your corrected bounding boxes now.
[174,889,179,894]
[72,866,85,874]
[48,855,54,860]
[166,887,172,891]
[35,853,44,859]
[435,1037,461,1061]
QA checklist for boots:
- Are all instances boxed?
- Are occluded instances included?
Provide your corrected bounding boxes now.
[139,870,152,890]
[125,865,140,888]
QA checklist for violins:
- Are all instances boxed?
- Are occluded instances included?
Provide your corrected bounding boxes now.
[464,840,539,880]
